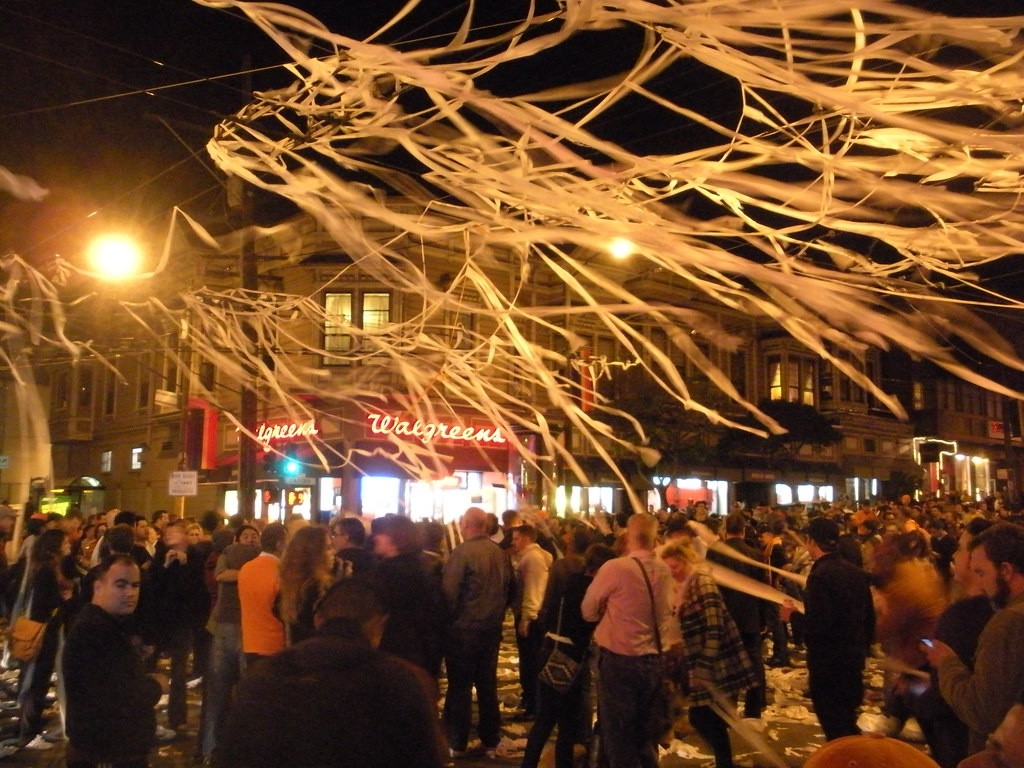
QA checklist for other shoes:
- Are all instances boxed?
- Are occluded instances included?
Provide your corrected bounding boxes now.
[448,748,463,757]
[24,735,54,750]
[767,655,791,667]
[479,741,495,755]
[177,721,190,730]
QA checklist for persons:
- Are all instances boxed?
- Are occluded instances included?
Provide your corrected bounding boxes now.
[779,518,874,741]
[211,575,448,768]
[0,492,1024,768]
[583,513,672,768]
[921,523,1024,750]
[660,539,760,768]
[62,554,167,768]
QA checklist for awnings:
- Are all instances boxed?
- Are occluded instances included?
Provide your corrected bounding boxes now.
[200,447,326,479]
[644,451,846,486]
[843,454,928,482]
[300,439,630,488]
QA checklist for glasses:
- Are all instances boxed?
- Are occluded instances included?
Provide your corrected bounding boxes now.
[331,532,346,539]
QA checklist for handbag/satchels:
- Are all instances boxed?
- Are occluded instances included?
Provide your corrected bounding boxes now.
[537,649,581,695]
[649,664,683,704]
[10,616,47,658]
